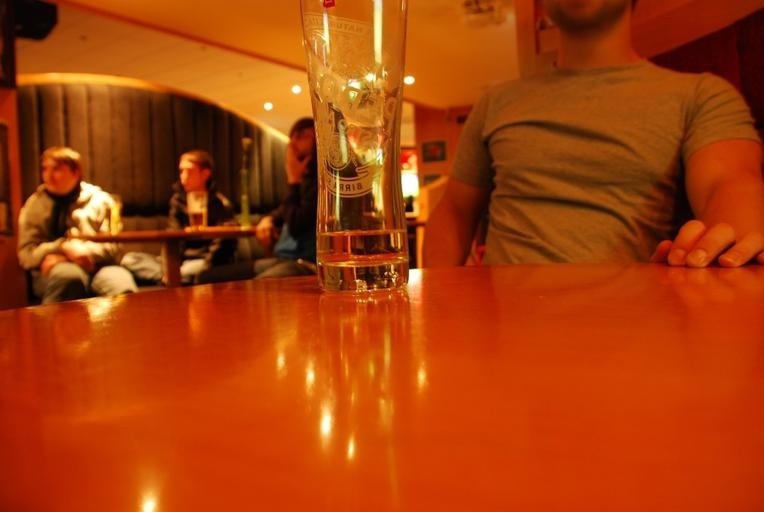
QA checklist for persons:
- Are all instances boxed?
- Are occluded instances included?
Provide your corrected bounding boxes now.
[421,1,764,269]
[16,146,136,304]
[403,197,416,234]
[253,118,373,280]
[119,148,242,286]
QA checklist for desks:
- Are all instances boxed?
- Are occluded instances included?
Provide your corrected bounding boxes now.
[65,225,256,287]
[0,262,764,512]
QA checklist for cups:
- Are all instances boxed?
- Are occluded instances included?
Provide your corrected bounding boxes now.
[185,191,207,231]
[299,0,411,300]
